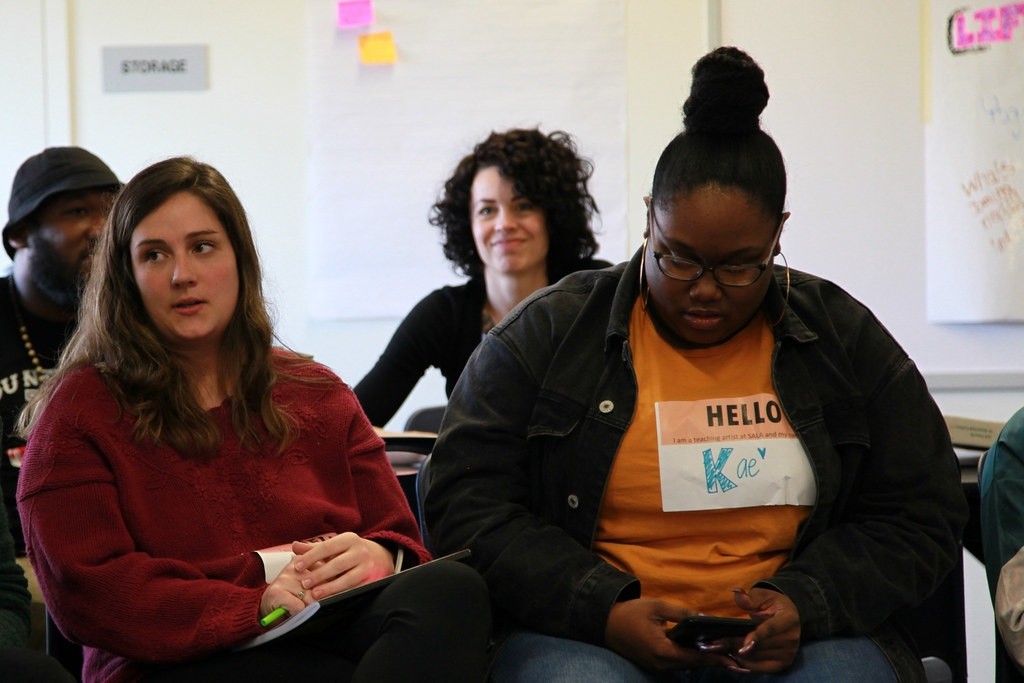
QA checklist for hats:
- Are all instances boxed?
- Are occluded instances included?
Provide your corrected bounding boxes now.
[2,146,125,261]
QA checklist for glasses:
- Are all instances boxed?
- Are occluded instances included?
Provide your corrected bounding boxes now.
[649,199,785,288]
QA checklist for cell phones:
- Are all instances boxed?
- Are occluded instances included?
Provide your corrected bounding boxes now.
[667,616,758,651]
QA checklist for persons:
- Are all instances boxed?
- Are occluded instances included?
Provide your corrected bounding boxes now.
[354,129,617,429]
[15,156,494,683]
[0,144,132,682]
[414,45,974,683]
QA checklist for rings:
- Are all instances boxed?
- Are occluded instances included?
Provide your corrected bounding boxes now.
[296,588,306,598]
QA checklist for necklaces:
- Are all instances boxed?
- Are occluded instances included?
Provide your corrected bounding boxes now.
[19,319,46,381]
